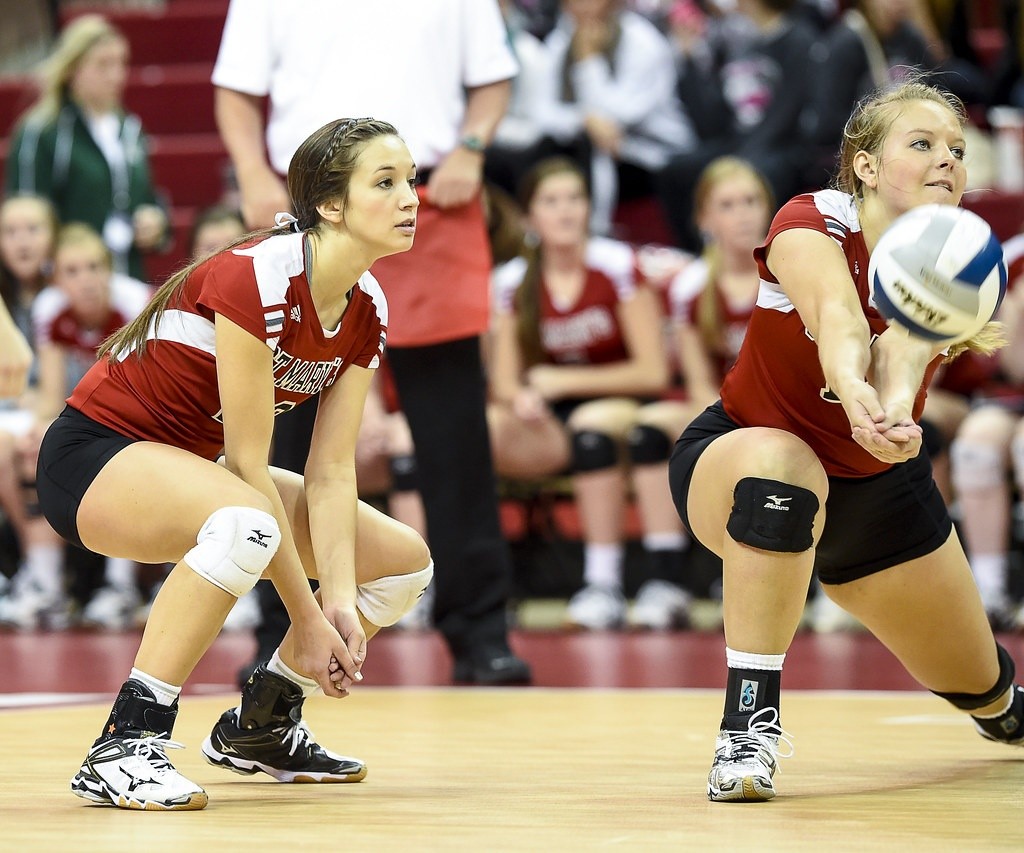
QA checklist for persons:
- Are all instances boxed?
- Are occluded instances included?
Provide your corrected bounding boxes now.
[0,15,435,631]
[211,0,538,686]
[36,120,437,810]
[485,0,1024,630]
[671,62,1024,802]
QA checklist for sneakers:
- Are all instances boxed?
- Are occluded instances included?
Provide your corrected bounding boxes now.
[70,731,208,811]
[706,707,795,801]
[969,685,1024,751]
[200,707,367,784]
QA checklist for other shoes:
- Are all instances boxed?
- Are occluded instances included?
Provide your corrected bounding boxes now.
[624,577,694,631]
[82,583,144,628]
[455,650,534,685]
[565,582,625,630]
[0,574,72,627]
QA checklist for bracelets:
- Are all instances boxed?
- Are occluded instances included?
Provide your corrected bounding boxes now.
[459,137,487,154]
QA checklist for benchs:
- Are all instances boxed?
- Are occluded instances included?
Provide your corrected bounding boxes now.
[0,0,1024,692]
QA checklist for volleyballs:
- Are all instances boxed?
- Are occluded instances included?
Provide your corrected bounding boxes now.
[866,202,1009,347]
